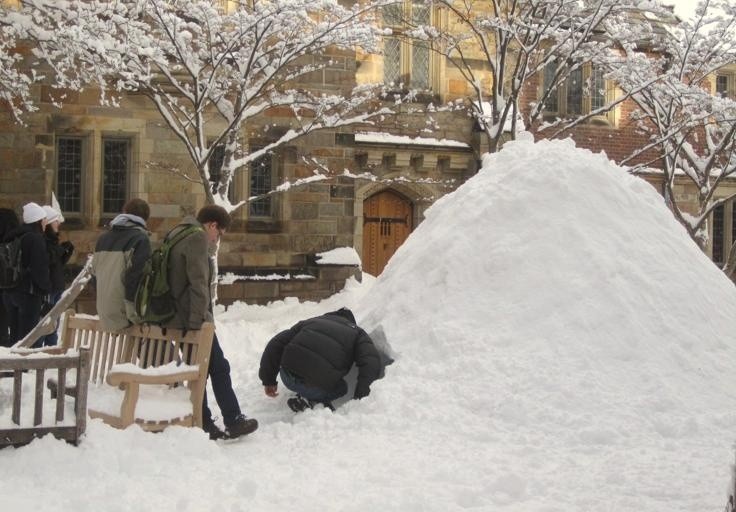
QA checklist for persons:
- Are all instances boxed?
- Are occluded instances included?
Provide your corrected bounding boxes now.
[0,202,85,373]
[89,200,182,390]
[158,203,259,441]
[258,305,381,413]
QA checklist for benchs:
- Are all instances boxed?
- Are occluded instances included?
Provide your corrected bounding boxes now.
[12,308,215,432]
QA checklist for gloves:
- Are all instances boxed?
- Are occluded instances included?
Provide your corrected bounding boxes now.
[61,240,74,256]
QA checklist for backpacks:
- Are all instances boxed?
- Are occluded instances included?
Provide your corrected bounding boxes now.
[133,225,207,328]
[0,232,35,289]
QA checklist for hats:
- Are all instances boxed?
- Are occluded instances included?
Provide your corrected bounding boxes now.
[21,202,48,224]
[40,204,60,225]
[122,198,150,221]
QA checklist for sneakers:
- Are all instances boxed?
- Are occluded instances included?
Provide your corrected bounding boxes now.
[225,414,260,439]
[201,421,229,441]
[284,396,336,414]
